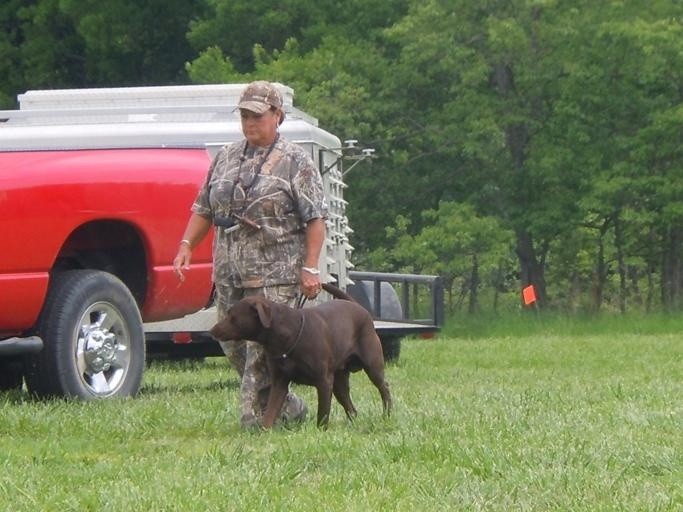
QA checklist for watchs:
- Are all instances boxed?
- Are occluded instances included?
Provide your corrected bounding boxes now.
[302,266,321,276]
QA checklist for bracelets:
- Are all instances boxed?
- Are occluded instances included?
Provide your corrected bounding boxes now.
[177,239,192,251]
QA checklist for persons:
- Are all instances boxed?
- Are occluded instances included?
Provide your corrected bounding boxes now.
[172,80,330,427]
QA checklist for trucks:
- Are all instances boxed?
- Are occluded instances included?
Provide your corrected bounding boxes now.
[0,82,445,372]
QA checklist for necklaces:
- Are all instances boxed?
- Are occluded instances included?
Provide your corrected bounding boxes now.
[228,132,280,202]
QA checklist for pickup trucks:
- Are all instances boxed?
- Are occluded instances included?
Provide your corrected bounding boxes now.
[0,145,218,402]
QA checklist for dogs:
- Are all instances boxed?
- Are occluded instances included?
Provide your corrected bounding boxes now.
[209,281,395,433]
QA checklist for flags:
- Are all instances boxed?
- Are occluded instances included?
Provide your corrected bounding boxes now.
[523,285,537,306]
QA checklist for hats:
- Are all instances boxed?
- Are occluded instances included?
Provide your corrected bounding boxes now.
[231,81,284,114]
